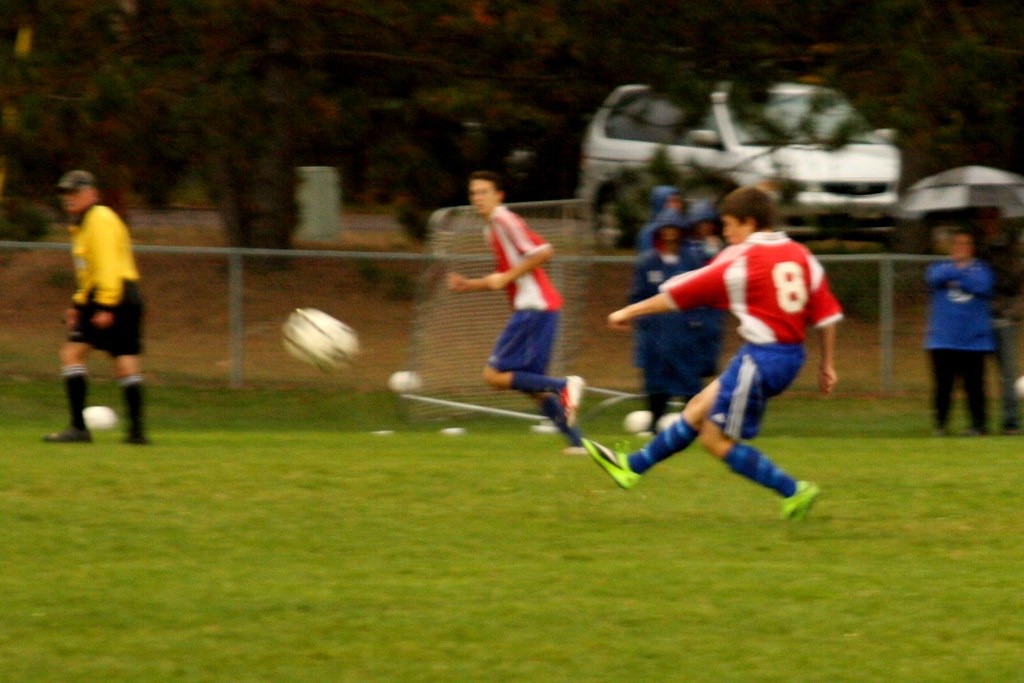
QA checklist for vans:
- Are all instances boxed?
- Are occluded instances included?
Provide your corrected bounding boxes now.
[578,75,907,250]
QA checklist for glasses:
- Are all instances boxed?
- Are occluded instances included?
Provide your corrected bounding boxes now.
[951,239,974,246]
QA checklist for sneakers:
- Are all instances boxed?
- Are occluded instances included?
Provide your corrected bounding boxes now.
[561,446,590,456]
[559,375,585,429]
[44,422,92,443]
[779,480,822,521]
[579,438,643,490]
[127,435,148,446]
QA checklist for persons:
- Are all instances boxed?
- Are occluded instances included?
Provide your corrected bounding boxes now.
[629,187,724,437]
[579,185,844,522]
[918,219,1024,436]
[41,169,149,445]
[450,170,590,456]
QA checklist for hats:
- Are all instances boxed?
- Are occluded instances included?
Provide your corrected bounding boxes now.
[55,170,97,190]
[651,186,682,209]
[687,200,719,223]
[638,208,686,248]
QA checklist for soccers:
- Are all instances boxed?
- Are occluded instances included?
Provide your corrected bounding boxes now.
[284,305,360,372]
